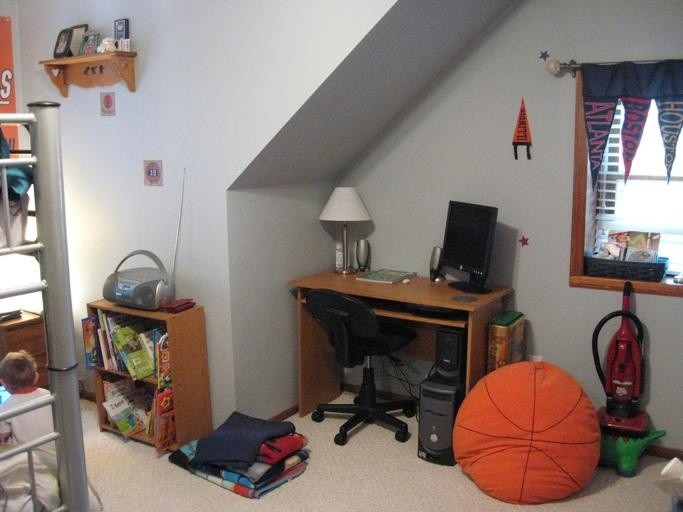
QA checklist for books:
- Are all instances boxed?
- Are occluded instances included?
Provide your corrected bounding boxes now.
[353,267,416,285]
[81,311,170,445]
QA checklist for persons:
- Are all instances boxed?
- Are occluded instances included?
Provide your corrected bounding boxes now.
[0,348,55,454]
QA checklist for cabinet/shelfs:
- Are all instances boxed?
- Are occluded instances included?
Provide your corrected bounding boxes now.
[87,304,212,453]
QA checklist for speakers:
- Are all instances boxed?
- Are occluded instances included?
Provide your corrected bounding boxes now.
[356,239,368,271]
[435,325,466,383]
[429,245,442,282]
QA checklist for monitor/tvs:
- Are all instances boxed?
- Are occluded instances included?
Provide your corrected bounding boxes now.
[436,199,498,294]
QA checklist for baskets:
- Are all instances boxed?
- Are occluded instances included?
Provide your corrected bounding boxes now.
[584,256,669,283]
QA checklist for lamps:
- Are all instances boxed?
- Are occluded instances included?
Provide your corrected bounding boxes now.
[317,186,372,273]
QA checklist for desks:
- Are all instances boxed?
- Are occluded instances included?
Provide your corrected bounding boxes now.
[289,267,513,417]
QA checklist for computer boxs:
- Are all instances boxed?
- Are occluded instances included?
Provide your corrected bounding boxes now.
[417,373,465,466]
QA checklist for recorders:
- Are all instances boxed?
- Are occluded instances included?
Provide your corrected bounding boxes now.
[103,167,186,311]
